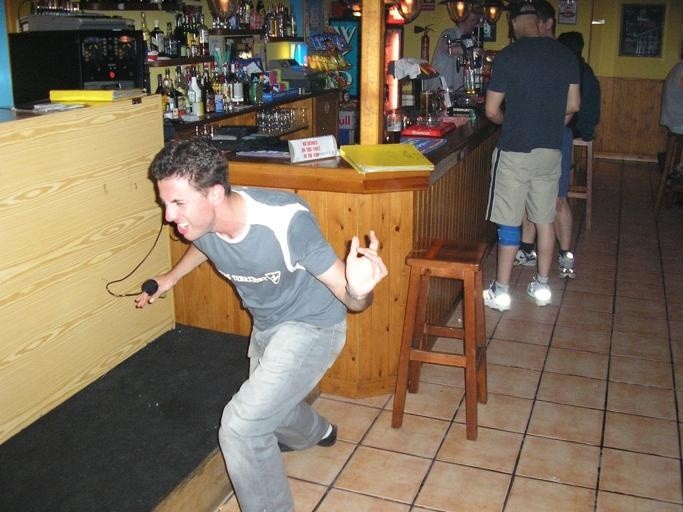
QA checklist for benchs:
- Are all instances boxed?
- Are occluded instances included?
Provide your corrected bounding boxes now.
[0,323,250,511]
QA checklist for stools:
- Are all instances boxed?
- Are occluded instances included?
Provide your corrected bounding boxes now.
[389,239,492,443]
[651,131,682,218]
[564,137,592,232]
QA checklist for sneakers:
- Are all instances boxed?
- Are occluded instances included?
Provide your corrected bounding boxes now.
[526,276,552,307]
[276,422,338,453]
[557,252,577,280]
[483,279,511,312]
[512,249,538,268]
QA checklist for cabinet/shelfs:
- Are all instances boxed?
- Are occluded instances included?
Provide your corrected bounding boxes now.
[80,1,305,68]
[277,92,337,144]
[168,93,308,139]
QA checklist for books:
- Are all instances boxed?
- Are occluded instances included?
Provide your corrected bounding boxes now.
[340,144,434,174]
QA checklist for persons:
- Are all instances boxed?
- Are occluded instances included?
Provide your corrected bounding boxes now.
[513,0,581,279]
[555,31,601,204]
[482,1,573,311]
[134,137,389,512]
[659,61,683,186]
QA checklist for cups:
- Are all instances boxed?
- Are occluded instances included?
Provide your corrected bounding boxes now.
[386,113,402,144]
[419,90,441,120]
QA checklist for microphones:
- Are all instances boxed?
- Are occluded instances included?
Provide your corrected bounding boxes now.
[141,279,167,298]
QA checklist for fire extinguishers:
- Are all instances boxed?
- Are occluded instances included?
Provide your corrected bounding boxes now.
[414,24,436,65]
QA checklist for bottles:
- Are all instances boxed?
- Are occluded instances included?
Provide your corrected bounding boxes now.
[464,54,483,96]
[140,0,309,142]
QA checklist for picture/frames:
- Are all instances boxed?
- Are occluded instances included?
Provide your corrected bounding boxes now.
[617,3,664,58]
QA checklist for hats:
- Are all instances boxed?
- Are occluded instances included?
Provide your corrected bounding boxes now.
[509,1,538,20]
